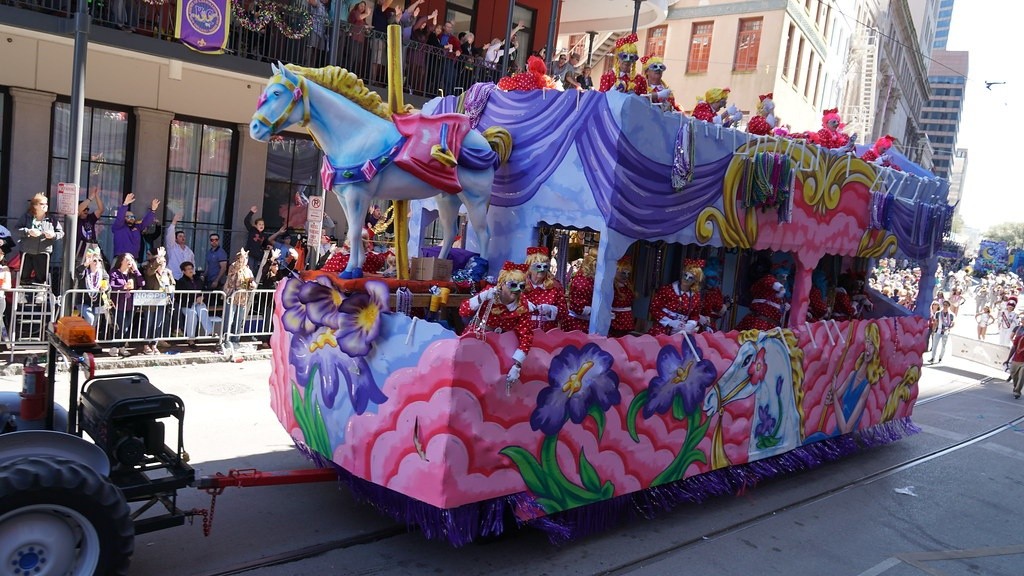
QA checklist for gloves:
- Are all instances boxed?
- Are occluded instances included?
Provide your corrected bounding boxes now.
[527,290,872,334]
[882,155,888,160]
[157,246,166,258]
[727,104,742,122]
[480,287,496,301]
[763,98,773,109]
[250,280,256,289]
[657,89,671,100]
[241,247,298,261]
[507,365,521,385]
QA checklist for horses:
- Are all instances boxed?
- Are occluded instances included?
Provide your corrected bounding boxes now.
[248,59,514,281]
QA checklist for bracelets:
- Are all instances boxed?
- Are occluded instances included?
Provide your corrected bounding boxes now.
[514,362,522,368]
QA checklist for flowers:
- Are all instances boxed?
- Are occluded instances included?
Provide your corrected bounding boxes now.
[279,264,390,359]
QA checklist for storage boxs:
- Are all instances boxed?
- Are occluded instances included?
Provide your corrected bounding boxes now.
[198,316,222,342]
[243,314,263,333]
[410,257,453,281]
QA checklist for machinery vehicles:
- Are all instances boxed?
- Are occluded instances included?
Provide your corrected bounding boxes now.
[0,309,198,575]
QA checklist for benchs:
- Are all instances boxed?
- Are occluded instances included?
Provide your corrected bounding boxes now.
[136,305,222,313]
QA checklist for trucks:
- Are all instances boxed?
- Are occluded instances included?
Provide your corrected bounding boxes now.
[937,235,1023,284]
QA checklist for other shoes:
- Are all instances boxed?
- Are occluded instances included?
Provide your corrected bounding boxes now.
[36,292,44,304]
[17,292,27,304]
[929,358,934,363]
[109,331,271,358]
[1006,363,1020,400]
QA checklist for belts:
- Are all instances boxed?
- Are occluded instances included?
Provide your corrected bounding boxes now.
[476,318,505,335]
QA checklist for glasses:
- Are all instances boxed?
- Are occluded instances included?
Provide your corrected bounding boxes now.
[125,215,135,219]
[210,239,218,242]
[560,57,566,60]
[83,208,89,211]
[94,259,102,262]
[286,237,291,239]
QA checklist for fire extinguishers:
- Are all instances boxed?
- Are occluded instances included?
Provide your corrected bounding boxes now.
[18,354,49,421]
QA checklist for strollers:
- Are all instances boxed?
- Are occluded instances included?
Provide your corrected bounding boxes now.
[7,250,50,359]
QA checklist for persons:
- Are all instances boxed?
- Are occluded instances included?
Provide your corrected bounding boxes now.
[244,203,268,276]
[262,248,300,350]
[265,224,297,270]
[832,269,873,322]
[861,135,900,171]
[551,54,567,79]
[772,261,831,323]
[817,107,850,150]
[868,253,1024,399]
[458,260,533,386]
[366,203,383,228]
[562,247,599,335]
[745,93,778,137]
[522,246,569,332]
[700,269,734,333]
[576,66,593,90]
[0,187,257,358]
[100,0,550,96]
[560,51,581,90]
[608,256,636,340]
[735,274,792,331]
[692,87,742,129]
[599,33,647,96]
[639,53,682,113]
[647,257,705,337]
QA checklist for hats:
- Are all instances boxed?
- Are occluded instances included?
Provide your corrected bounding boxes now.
[572,53,581,60]
